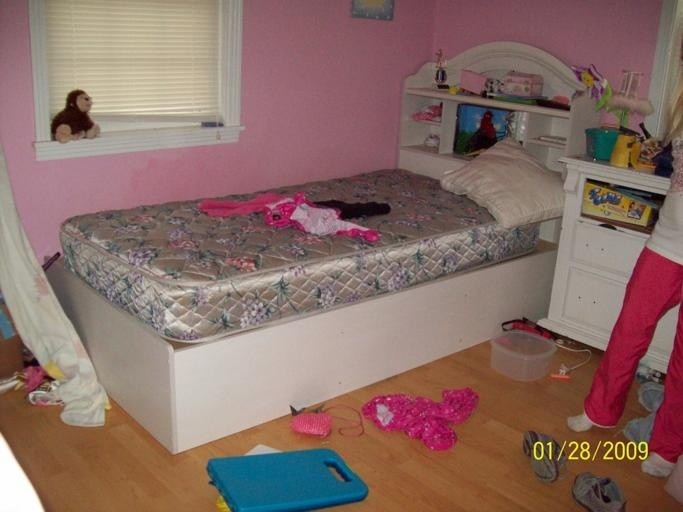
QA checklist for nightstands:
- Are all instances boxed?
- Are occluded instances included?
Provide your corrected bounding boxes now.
[537,146,681,381]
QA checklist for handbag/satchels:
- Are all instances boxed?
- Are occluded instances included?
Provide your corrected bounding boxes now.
[291,413,332,439]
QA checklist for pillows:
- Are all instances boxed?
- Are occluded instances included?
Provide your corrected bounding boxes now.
[436,136,578,230]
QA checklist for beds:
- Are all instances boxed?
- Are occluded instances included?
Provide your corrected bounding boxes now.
[43,37,604,459]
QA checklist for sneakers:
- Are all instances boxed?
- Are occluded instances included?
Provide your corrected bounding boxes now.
[571,472,627,511]
[522,430,566,483]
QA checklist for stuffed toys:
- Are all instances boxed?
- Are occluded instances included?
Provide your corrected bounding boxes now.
[49,89,100,144]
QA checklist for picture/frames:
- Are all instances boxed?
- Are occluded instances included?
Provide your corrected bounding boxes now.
[450,100,518,162]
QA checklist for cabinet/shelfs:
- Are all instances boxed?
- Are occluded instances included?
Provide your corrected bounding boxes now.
[399,85,570,179]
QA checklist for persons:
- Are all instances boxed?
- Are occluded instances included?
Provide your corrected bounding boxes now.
[567,2,683,477]
